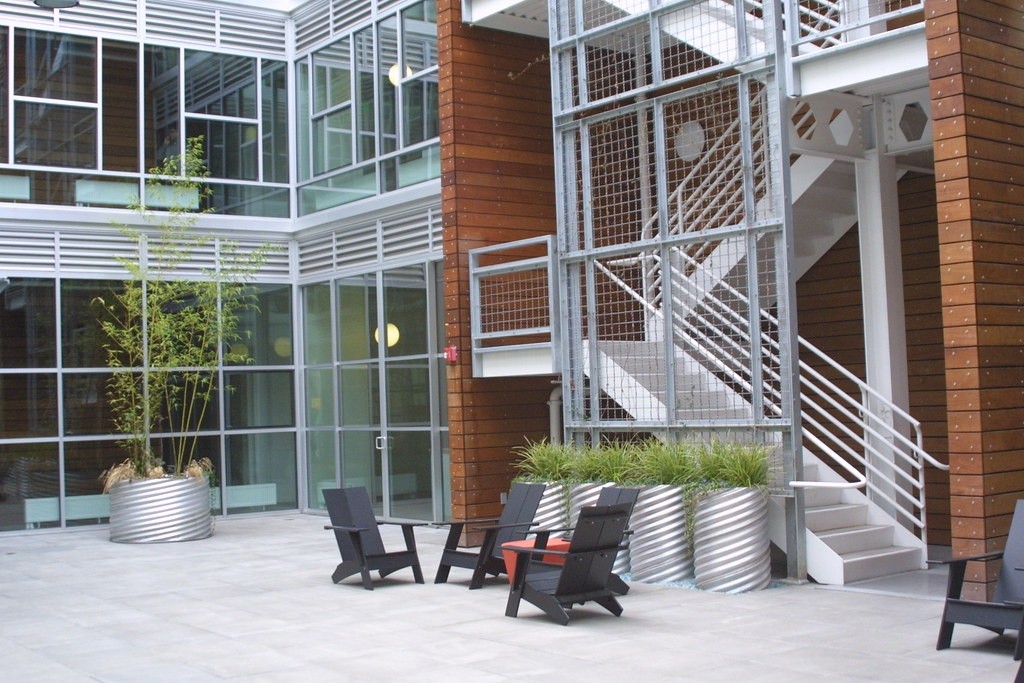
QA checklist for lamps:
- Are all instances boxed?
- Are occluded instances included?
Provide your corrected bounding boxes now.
[389,62,413,86]
[375,323,399,348]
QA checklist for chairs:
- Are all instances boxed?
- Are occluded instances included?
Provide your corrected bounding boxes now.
[322,487,429,590]
[431,483,547,591]
[495,502,633,626]
[561,487,641,596]
[925,498,1024,662]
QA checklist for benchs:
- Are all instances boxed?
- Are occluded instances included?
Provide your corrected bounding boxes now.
[23,483,277,530]
[74,180,199,210]
[0,174,30,203]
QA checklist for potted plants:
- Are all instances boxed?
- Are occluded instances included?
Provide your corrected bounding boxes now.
[505,434,783,594]
[91,135,285,544]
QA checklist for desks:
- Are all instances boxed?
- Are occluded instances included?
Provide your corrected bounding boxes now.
[500,538,570,586]
